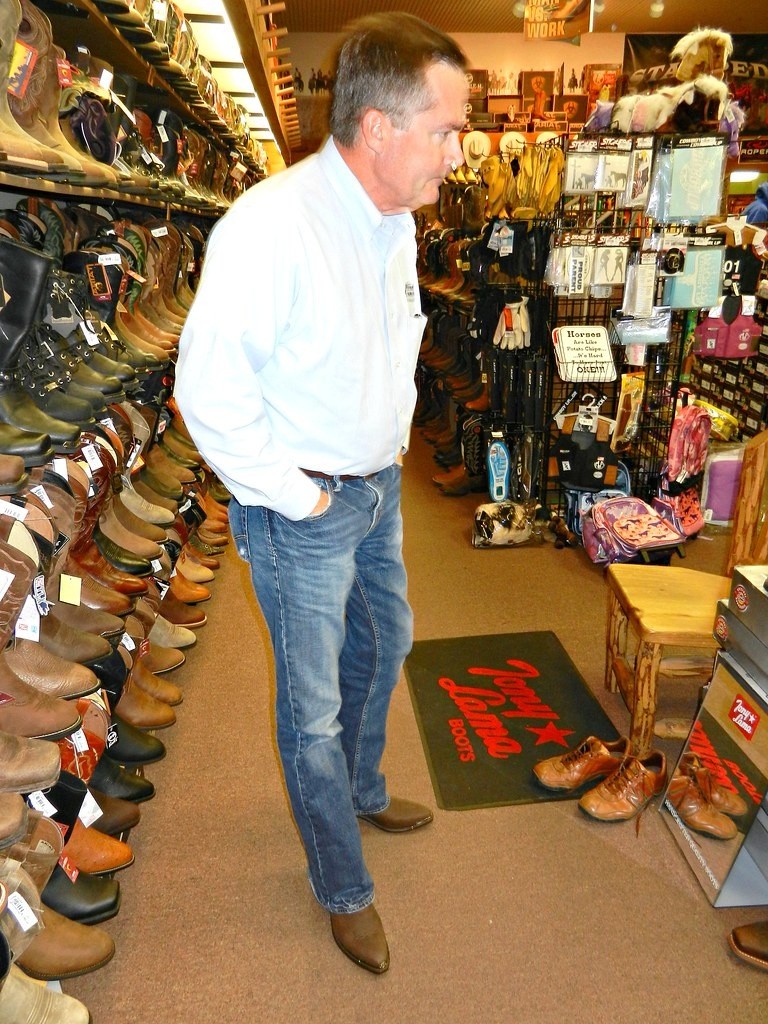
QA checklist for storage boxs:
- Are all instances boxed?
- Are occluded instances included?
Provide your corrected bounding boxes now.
[712,599,768,694]
[727,564,768,647]
[461,70,589,133]
[684,268,768,443]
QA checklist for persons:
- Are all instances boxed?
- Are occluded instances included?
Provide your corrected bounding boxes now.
[176,10,469,975]
[739,182,768,227]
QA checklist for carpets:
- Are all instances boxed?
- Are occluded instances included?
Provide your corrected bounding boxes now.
[403,630,626,810]
[684,707,768,835]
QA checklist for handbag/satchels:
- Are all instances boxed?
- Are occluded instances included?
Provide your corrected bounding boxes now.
[472,502,538,548]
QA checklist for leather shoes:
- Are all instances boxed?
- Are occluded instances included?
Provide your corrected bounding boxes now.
[728,921,768,973]
[330,902,390,975]
[357,795,434,834]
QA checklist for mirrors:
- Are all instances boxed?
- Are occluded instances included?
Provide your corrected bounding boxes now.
[657,664,768,906]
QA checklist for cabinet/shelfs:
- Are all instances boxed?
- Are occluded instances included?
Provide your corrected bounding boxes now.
[0,0,261,994]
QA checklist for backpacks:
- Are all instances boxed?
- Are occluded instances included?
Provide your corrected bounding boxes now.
[690,398,738,445]
[660,403,712,497]
[660,487,706,539]
[590,496,688,564]
[561,458,632,538]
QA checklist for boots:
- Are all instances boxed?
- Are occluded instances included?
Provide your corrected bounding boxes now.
[414,167,520,498]
[0,0,271,1024]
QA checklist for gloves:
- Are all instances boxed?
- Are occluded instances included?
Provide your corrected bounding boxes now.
[470,134,570,421]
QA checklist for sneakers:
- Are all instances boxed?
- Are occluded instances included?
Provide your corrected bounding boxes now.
[669,766,738,841]
[578,748,671,824]
[678,752,749,818]
[532,734,636,793]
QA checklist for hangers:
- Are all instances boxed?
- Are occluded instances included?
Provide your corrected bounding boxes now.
[554,393,617,435]
[571,414,596,449]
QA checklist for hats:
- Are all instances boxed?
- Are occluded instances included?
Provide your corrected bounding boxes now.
[536,132,559,145]
[463,129,491,169]
[499,132,527,154]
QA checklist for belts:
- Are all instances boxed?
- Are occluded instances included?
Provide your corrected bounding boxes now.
[299,467,381,482]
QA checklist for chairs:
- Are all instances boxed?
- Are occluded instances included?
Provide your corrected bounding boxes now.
[603,428,768,757]
